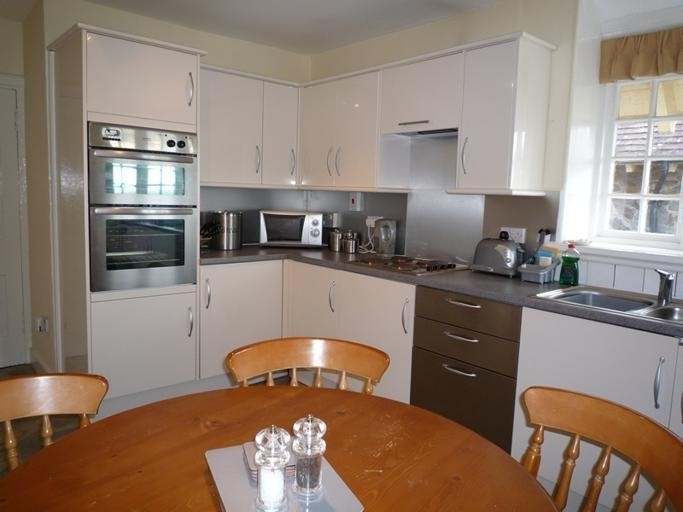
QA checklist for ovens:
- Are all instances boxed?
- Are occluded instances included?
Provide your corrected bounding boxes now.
[90,146,197,288]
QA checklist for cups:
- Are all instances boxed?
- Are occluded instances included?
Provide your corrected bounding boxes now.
[216,211,242,251]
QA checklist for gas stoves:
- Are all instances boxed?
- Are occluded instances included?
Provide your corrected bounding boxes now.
[353,252,467,276]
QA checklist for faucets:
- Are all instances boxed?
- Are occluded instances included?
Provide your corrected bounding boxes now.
[653,267,675,307]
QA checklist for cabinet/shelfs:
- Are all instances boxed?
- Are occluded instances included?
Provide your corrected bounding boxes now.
[445,30,557,196]
[59,282,196,405]
[341,266,416,409]
[49,20,209,130]
[406,279,524,463]
[197,258,281,382]
[303,63,411,193]
[263,75,299,189]
[199,64,263,188]
[504,299,680,512]
[280,253,344,390]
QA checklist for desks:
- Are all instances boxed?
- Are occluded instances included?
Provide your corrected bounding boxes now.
[1,385,558,512]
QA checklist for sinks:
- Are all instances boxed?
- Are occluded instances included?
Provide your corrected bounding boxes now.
[527,285,655,313]
[645,304,683,324]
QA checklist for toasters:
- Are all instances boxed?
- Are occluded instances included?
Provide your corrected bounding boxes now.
[469,229,524,279]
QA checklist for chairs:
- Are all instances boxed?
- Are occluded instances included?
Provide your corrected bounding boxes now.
[0,372,110,476]
[514,383,682,511]
[222,337,392,397]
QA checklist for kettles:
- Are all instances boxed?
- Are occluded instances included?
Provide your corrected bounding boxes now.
[372,219,397,254]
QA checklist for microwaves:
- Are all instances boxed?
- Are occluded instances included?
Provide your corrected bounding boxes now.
[260,248,324,261]
[257,210,323,248]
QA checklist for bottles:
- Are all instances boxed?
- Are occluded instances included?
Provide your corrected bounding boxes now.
[340,230,358,253]
[252,413,328,512]
[329,229,341,250]
